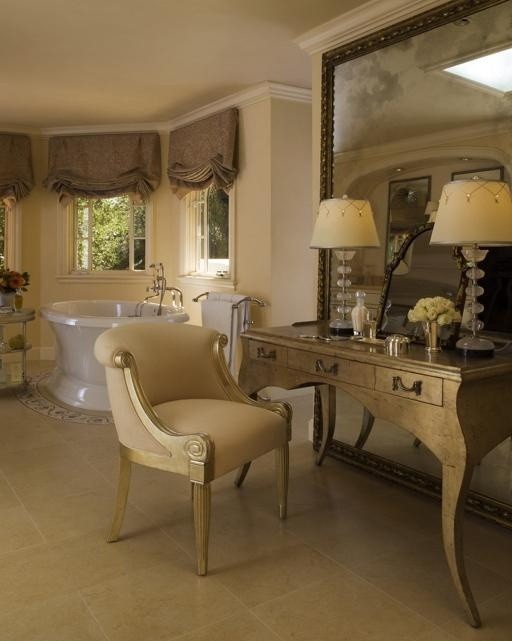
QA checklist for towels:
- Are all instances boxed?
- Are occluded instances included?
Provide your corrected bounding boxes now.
[199,300,239,369]
[207,291,251,333]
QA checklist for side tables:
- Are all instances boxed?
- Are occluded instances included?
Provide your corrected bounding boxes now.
[0,309,37,390]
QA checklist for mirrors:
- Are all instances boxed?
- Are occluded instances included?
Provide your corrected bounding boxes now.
[378,223,471,350]
[385,176,431,277]
[451,165,505,182]
[313,0,511,532]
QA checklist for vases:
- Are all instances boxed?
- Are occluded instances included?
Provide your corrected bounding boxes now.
[2,294,15,313]
[423,320,442,352]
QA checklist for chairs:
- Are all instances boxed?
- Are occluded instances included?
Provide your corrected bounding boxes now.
[94,322,293,577]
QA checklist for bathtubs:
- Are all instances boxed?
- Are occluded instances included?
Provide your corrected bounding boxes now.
[40,298,190,412]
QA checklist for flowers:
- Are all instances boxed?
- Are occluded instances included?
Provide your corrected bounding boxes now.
[0,267,31,293]
[406,296,461,324]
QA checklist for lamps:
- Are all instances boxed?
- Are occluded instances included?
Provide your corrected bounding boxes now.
[308,194,380,337]
[428,179,512,359]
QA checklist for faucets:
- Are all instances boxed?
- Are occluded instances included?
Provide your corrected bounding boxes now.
[143,262,183,316]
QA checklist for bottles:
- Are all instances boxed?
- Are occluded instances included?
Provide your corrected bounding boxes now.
[13,288,24,312]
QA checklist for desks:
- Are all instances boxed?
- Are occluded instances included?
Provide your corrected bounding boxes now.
[234,321,512,631]
